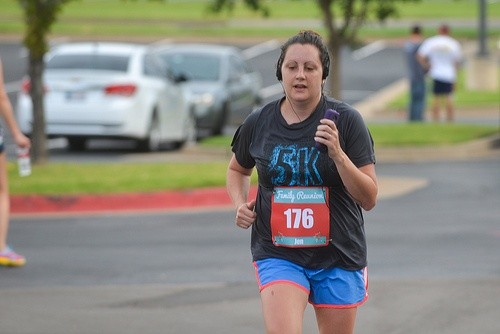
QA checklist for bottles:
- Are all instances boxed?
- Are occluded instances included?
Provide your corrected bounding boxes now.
[17,146,31,176]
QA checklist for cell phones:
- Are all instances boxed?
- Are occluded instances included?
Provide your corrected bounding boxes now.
[315,109,340,152]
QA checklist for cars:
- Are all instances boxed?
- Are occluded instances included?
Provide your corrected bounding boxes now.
[153,41,264,135]
[16,41,195,152]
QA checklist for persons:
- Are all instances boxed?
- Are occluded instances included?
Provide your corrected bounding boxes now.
[0,58,31,265]
[227,31,378,334]
[402,24,464,121]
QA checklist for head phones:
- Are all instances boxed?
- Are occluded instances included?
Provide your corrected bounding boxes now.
[276,55,331,80]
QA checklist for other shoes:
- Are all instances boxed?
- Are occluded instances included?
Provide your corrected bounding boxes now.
[0,247,26,266]
